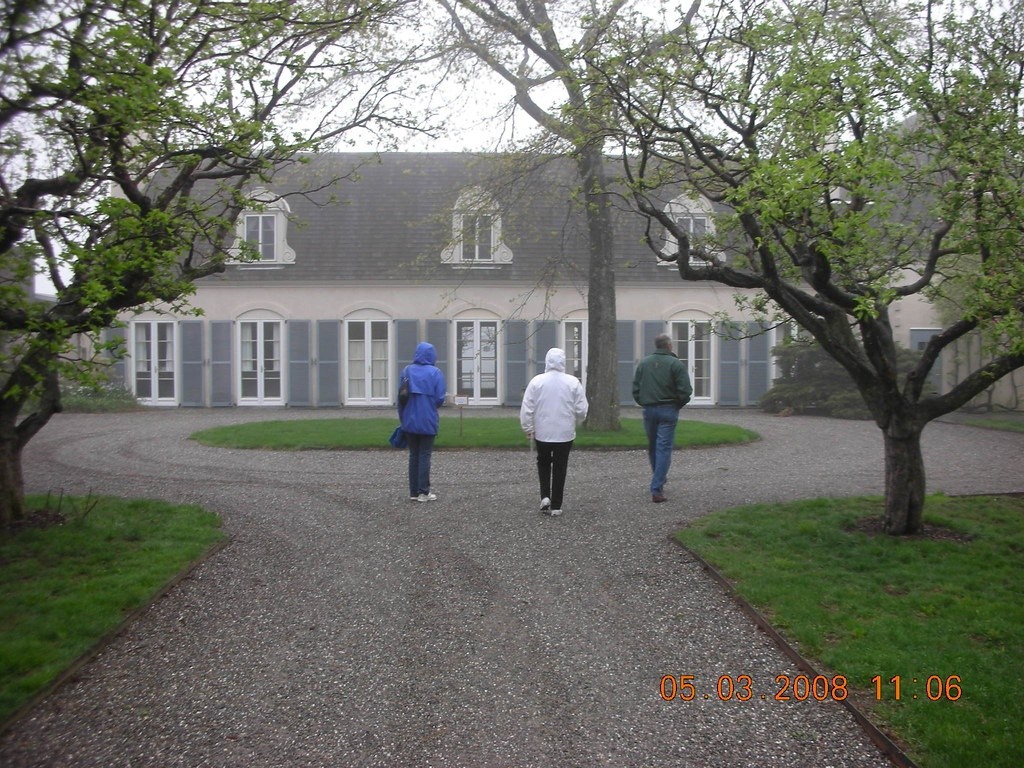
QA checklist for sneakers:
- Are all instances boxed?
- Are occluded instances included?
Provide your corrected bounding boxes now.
[410,497,418,500]
[540,497,551,511]
[550,509,562,516]
[653,495,666,503]
[418,493,437,502]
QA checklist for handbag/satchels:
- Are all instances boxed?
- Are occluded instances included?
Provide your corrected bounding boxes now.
[389,427,407,449]
[397,365,409,405]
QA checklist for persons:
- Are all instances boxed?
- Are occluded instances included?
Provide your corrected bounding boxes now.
[398,342,446,502]
[632,334,693,502]
[521,348,589,517]
